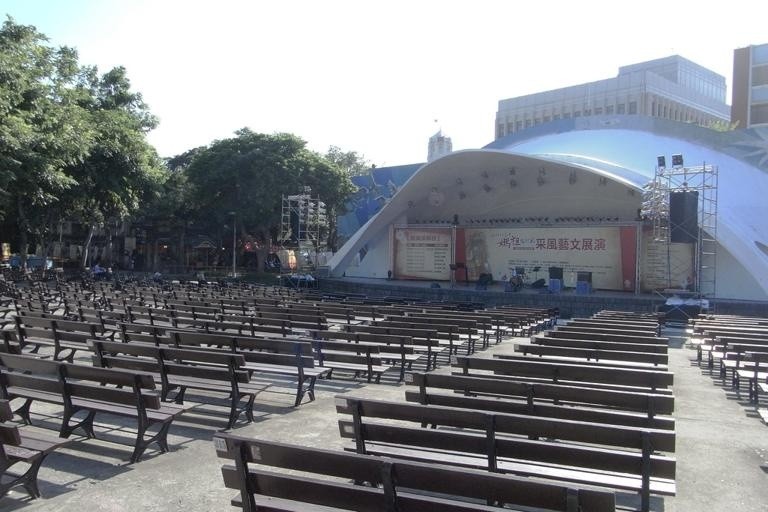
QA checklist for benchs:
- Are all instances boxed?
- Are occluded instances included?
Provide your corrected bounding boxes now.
[0,265,768,511]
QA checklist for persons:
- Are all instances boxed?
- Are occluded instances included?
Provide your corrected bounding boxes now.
[94,261,106,281]
[42,256,53,282]
[153,271,162,283]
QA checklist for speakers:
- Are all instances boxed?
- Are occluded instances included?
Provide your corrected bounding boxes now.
[475,282,487,290]
[577,272,592,282]
[669,191,699,243]
[504,283,513,292]
[430,282,441,288]
[532,279,546,288]
[549,267,563,279]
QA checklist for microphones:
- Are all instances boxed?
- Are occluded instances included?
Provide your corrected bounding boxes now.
[388,271,391,280]
